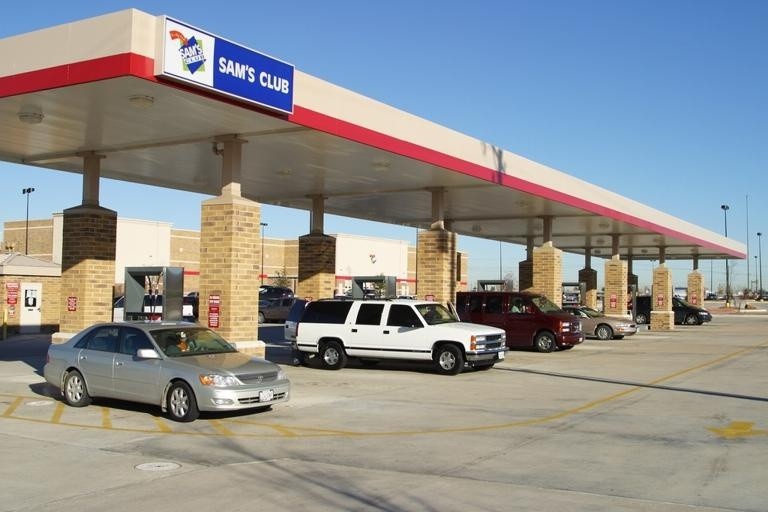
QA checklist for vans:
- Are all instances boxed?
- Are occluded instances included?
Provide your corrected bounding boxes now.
[457,288,584,354]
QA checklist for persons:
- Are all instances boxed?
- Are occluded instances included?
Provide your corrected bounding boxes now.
[510,299,522,314]
[25,290,36,307]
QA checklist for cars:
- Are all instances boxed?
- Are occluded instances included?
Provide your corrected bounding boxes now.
[182,282,308,342]
[563,303,638,342]
[42,319,291,422]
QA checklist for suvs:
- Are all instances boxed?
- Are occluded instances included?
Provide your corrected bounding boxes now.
[629,294,714,328]
[291,295,510,376]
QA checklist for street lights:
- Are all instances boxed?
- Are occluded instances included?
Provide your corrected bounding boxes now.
[756,232,761,298]
[22,186,34,255]
[260,222,267,288]
[753,255,757,293]
[720,203,730,306]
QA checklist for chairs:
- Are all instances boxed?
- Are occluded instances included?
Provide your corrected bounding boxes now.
[93,335,190,357]
